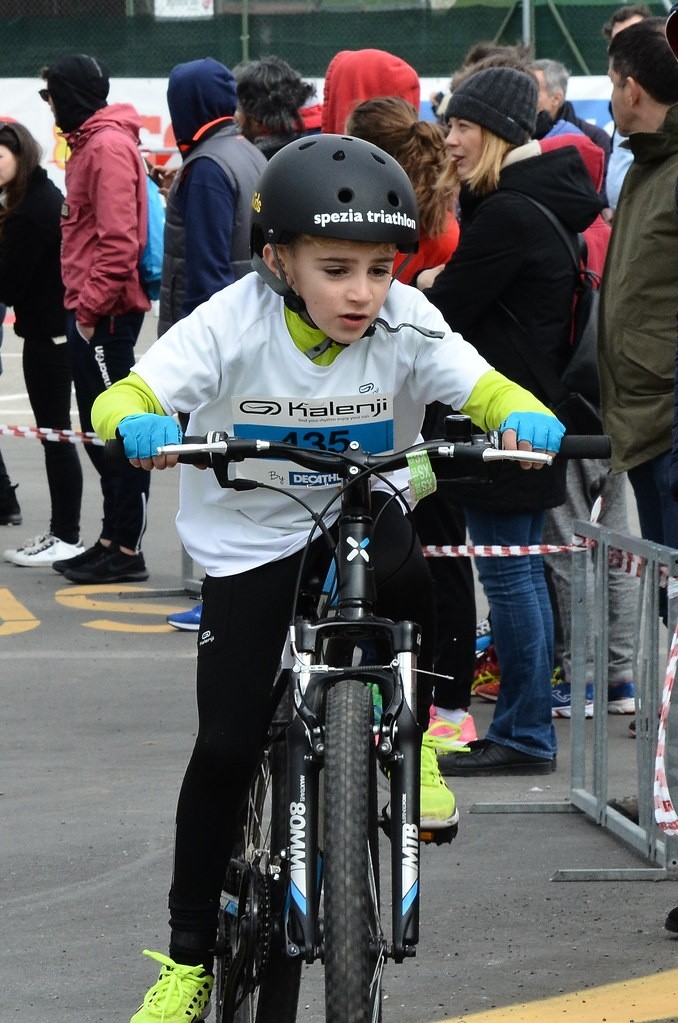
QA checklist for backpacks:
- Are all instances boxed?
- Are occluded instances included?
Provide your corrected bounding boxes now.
[136,172,168,299]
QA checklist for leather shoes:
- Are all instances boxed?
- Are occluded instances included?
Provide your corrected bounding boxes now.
[436,739,549,775]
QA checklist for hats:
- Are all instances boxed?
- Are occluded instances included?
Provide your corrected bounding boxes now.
[445,67,537,146]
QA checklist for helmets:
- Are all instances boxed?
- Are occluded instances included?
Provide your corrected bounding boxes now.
[250,134,420,261]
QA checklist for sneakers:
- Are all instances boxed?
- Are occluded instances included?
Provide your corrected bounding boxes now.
[479,667,566,703]
[377,736,459,828]
[166,605,201,630]
[10,538,85,567]
[52,540,111,572]
[64,542,150,583]
[130,966,215,1023]
[4,535,83,561]
[603,682,645,715]
[550,681,600,720]
[375,705,478,744]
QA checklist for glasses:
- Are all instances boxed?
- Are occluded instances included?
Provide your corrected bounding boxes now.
[38,89,50,101]
[0,122,18,143]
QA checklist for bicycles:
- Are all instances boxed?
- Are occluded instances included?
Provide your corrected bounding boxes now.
[106,412,613,1023]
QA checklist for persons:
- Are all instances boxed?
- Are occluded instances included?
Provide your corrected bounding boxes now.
[42,54,152,586]
[0,305,23,526]
[234,48,476,747]
[167,58,268,630]
[0,124,83,567]
[92,134,566,1023]
[438,4,678,776]
